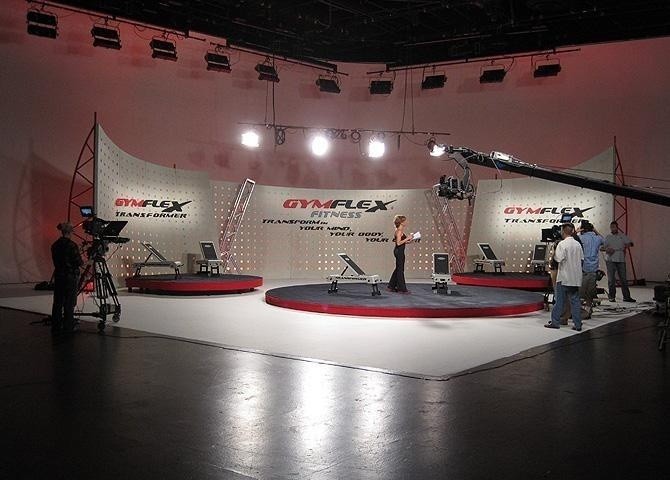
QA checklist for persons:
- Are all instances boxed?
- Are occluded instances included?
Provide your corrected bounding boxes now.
[389,214,414,291]
[49,222,85,339]
[544,219,636,332]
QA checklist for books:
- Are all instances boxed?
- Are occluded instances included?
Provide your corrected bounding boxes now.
[408,231,423,241]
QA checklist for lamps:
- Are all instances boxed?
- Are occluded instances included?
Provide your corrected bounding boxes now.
[366,48,581,95]
[205,34,349,94]
[25,0,206,62]
[236,52,451,158]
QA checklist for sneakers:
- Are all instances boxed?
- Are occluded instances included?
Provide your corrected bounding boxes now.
[573,326,581,330]
[623,298,636,302]
[609,297,615,301]
[545,323,560,329]
